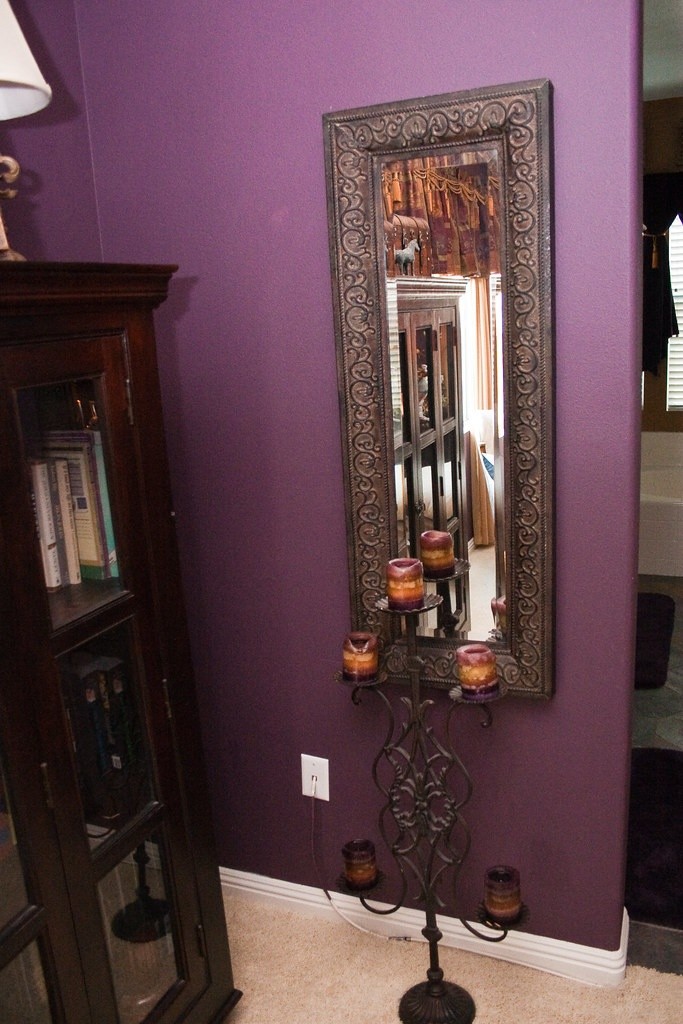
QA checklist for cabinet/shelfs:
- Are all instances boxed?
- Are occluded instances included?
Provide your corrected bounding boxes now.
[387,279,471,639]
[0,255,244,1024]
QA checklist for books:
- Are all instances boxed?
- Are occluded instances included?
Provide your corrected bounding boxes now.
[26,428,119,588]
[58,653,141,824]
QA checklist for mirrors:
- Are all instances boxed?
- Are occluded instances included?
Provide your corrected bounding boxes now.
[322,78,558,704]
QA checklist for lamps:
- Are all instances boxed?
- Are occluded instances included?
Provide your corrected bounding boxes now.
[0,0,54,263]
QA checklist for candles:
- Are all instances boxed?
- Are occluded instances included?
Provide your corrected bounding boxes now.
[386,558,425,610]
[455,640,500,700]
[342,839,378,888]
[420,530,457,578]
[484,866,521,915]
[342,632,381,683]
[492,595,509,642]
